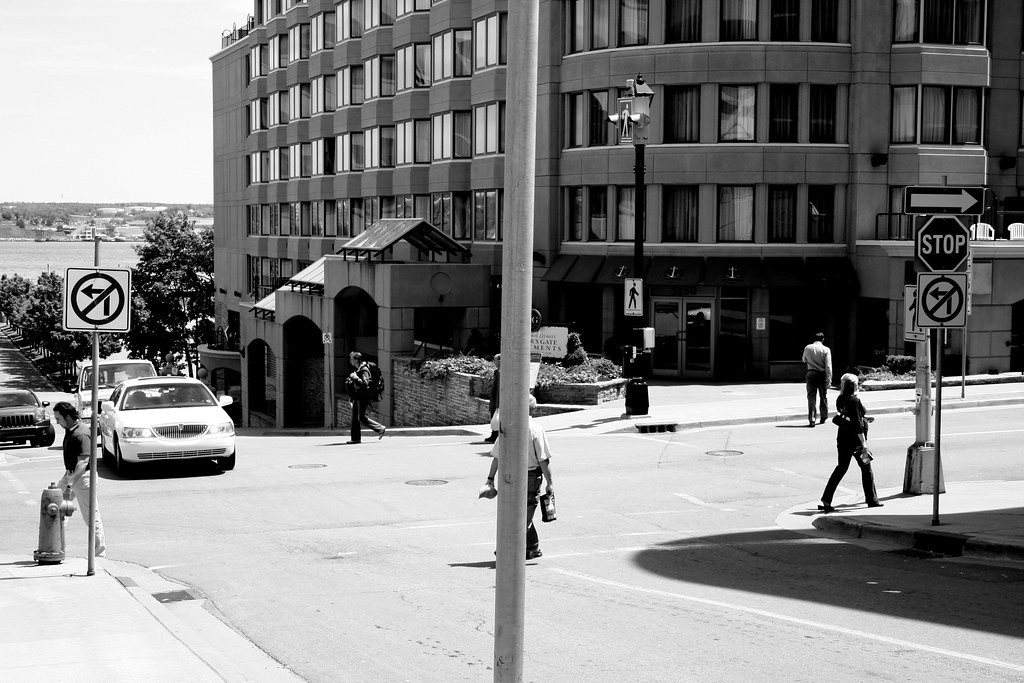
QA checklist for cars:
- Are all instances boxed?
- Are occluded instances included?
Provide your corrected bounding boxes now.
[0,389,53,447]
[75,357,235,475]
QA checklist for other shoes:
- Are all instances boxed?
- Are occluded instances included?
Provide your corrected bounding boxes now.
[811,422,815,427]
[485,436,497,442]
[348,441,362,444]
[821,417,827,424]
[525,550,542,560]
[379,425,386,440]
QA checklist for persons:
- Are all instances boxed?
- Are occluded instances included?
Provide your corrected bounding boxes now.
[802,333,832,427]
[484,393,554,560]
[346,351,386,444]
[52,401,107,558]
[484,354,501,444]
[821,373,884,513]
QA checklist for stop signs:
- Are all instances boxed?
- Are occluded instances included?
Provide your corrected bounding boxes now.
[911,213,973,275]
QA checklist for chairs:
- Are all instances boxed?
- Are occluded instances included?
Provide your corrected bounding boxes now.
[970,222,996,241]
[130,391,153,408]
[1008,223,1024,240]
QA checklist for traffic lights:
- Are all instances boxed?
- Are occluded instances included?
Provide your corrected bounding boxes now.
[608,95,649,147]
[633,327,656,348]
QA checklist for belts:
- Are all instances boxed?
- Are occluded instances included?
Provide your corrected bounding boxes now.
[70,469,74,473]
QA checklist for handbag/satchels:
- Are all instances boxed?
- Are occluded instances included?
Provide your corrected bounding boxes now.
[489,408,501,431]
[345,369,368,398]
[832,396,858,429]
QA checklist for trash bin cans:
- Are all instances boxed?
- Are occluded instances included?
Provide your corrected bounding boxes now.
[625,378,649,415]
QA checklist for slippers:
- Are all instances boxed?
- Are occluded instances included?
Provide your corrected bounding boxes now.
[867,502,884,507]
[818,504,834,512]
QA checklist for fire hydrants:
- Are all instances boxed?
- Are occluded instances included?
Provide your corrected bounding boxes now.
[34,483,79,563]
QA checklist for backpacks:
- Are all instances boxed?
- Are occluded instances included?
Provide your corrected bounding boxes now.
[361,361,385,394]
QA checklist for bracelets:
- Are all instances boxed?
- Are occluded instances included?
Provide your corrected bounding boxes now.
[66,484,71,489]
[488,477,494,480]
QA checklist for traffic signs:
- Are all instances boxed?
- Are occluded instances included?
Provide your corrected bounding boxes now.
[901,185,987,218]
